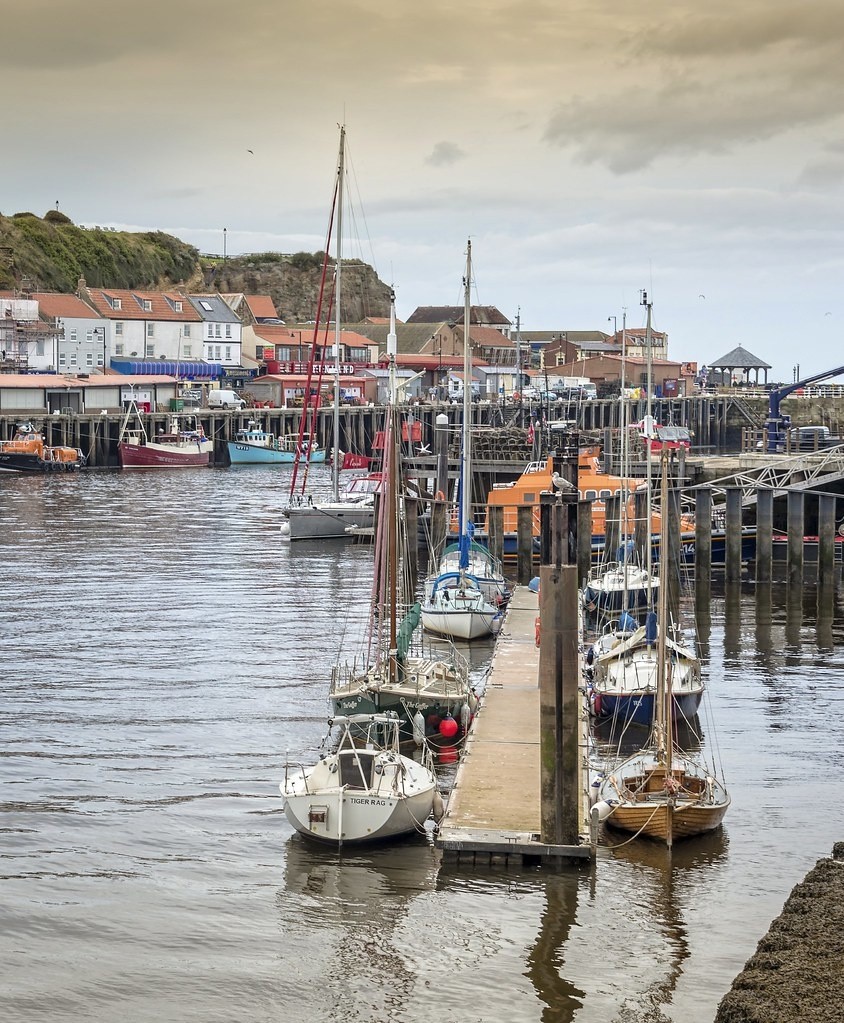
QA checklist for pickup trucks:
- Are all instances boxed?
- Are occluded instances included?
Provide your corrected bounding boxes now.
[753,422,844,454]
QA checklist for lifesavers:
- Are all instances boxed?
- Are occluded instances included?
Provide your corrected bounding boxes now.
[535,616,541,648]
[435,490,444,503]
[538,582,541,607]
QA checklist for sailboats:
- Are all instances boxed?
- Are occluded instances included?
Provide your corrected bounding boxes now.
[0,121,761,856]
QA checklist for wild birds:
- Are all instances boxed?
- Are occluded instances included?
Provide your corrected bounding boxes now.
[698,295,705,299]
[248,150,254,154]
